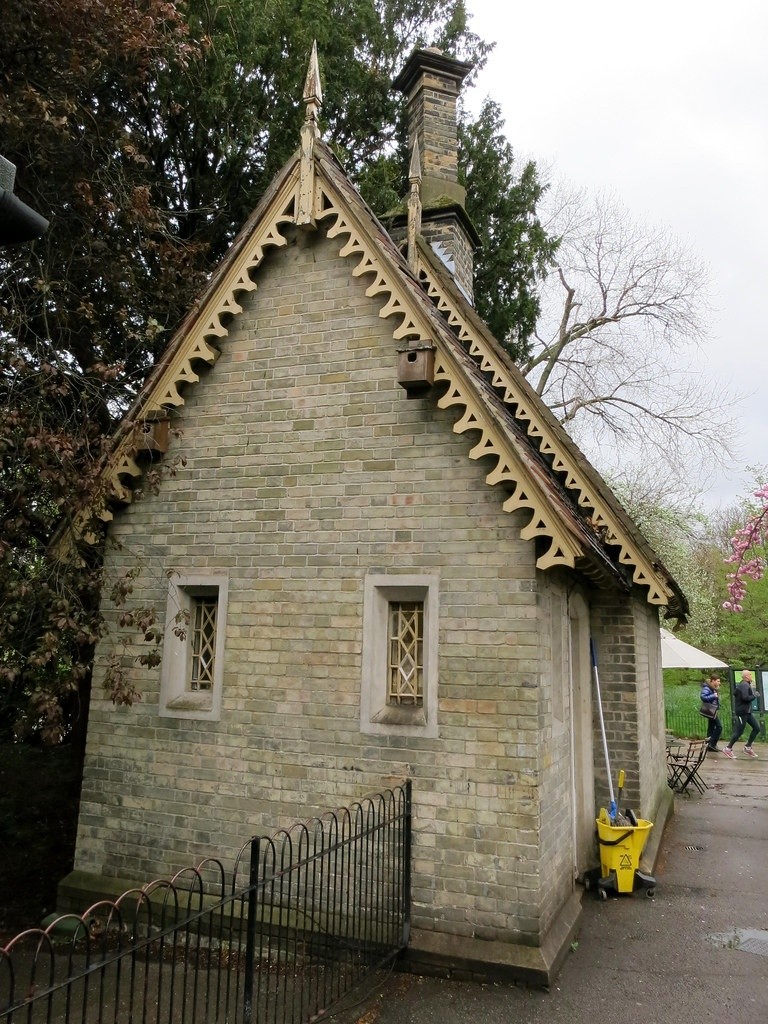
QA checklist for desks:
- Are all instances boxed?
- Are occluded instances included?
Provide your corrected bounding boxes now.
[665,741,686,792]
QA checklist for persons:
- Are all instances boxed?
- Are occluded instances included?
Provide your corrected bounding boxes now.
[722,668,760,759]
[701,674,723,752]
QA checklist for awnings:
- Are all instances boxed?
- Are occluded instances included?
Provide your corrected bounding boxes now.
[660,628,729,669]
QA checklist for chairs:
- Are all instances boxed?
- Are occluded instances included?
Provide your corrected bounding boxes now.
[667,740,706,799]
[669,737,698,783]
[680,736,712,790]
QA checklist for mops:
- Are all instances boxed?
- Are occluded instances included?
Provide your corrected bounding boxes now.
[588,634,634,828]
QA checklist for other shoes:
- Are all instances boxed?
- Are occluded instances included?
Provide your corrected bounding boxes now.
[708,744,722,752]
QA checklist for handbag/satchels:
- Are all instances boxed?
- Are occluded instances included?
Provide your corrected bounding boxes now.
[699,701,719,719]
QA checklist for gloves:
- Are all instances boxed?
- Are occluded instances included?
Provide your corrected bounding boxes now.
[753,691,760,697]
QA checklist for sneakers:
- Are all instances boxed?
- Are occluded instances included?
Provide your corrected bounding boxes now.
[723,747,737,758]
[743,746,758,758]
